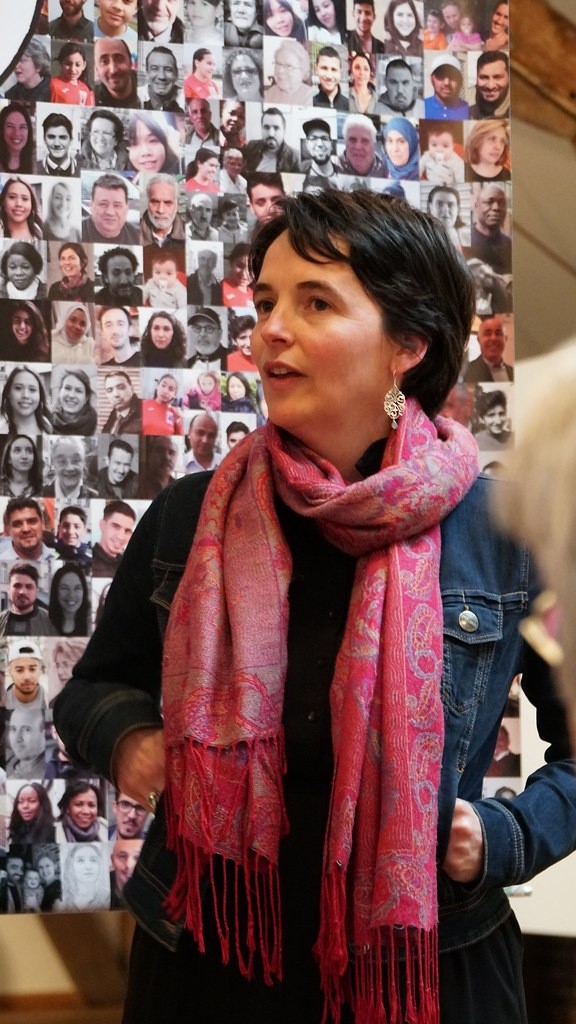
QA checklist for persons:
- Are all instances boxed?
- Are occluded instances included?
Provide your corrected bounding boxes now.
[53,190,576,1023]
[96,306,140,366]
[378,59,425,118]
[137,46,183,114]
[50,43,95,106]
[186,194,220,241]
[264,39,314,106]
[0,364,53,435]
[81,110,136,172]
[100,372,142,433]
[98,440,138,498]
[0,1,532,915]
[54,507,89,559]
[188,307,227,369]
[185,415,222,474]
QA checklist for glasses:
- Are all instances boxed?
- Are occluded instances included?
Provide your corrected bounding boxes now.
[308,136,331,143]
[273,61,302,71]
[18,58,33,64]
[11,317,34,325]
[117,800,148,816]
[191,325,219,333]
[230,68,260,77]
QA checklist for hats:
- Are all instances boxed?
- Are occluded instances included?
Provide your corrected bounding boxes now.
[303,118,330,137]
[188,308,221,329]
[8,640,42,662]
[430,54,461,76]
